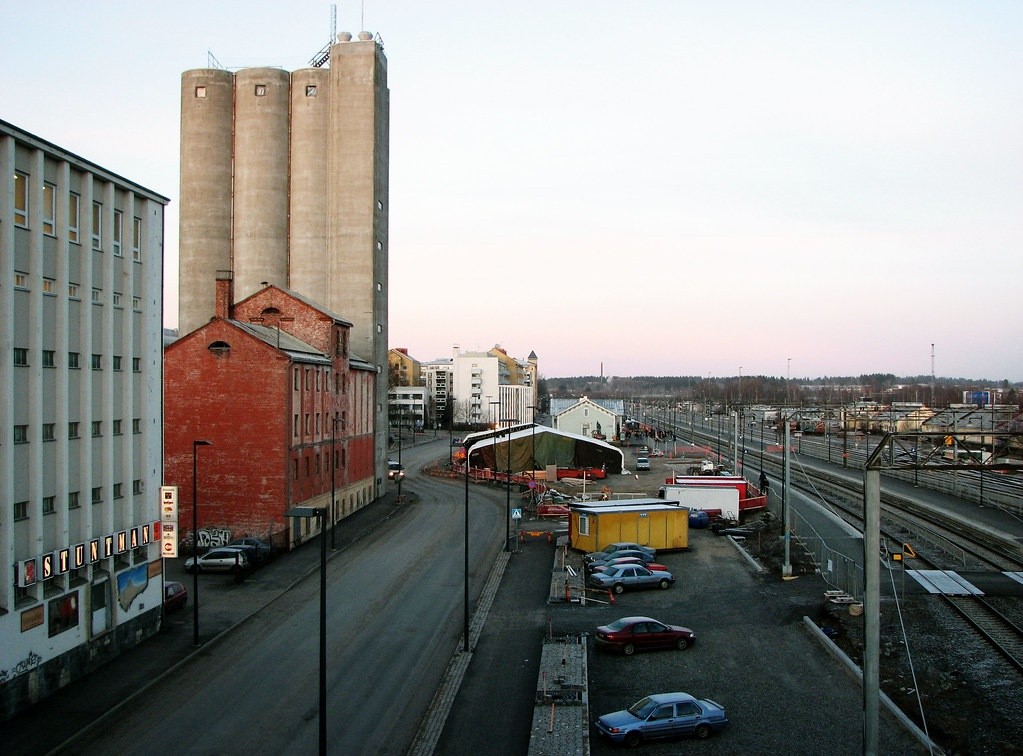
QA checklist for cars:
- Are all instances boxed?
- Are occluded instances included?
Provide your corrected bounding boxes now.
[594,616,696,656]
[226,538,278,563]
[595,692,729,746]
[585,543,675,595]
[538,503,570,516]
[183,548,250,573]
[837,429,855,438]
[636,458,650,471]
[388,461,405,479]
[164,581,187,613]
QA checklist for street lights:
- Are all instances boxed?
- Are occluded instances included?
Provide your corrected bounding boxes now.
[412,396,416,443]
[450,397,456,466]
[454,444,469,651]
[332,418,345,548]
[718,409,777,473]
[526,405,536,504]
[781,416,803,536]
[284,506,326,756]
[192,439,212,647]
[489,401,520,552]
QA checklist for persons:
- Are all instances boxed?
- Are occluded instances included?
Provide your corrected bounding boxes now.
[759,471,767,493]
[597,484,610,501]
